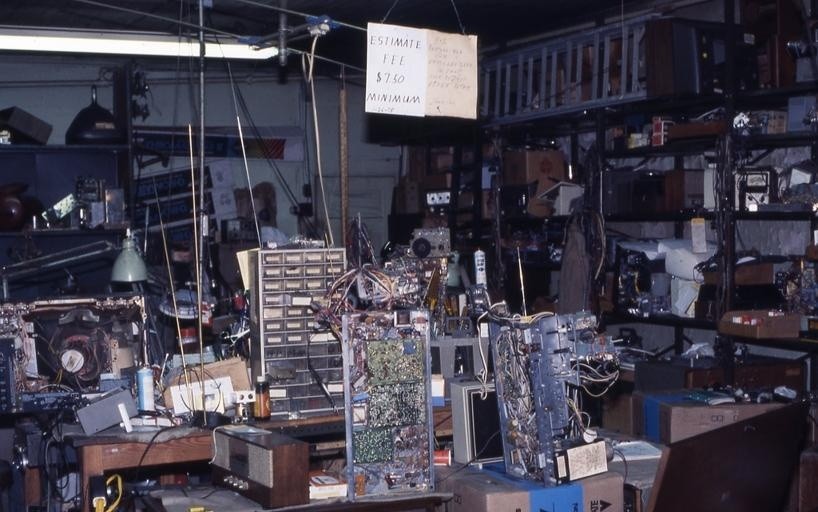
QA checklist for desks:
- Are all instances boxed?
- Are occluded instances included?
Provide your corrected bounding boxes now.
[70,413,344,512]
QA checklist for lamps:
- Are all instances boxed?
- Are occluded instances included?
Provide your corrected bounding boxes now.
[112,226,148,282]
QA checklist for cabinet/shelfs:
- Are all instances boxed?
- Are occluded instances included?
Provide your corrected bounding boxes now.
[446,81,818,391]
[0,63,135,237]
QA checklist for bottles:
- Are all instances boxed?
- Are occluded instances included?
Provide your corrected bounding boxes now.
[254,376,271,419]
[138,365,155,418]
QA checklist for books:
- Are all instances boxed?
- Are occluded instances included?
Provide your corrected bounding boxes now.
[689,389,736,407]
[308,470,348,500]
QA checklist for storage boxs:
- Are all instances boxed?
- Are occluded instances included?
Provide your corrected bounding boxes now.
[633,386,787,444]
[434,465,624,512]
